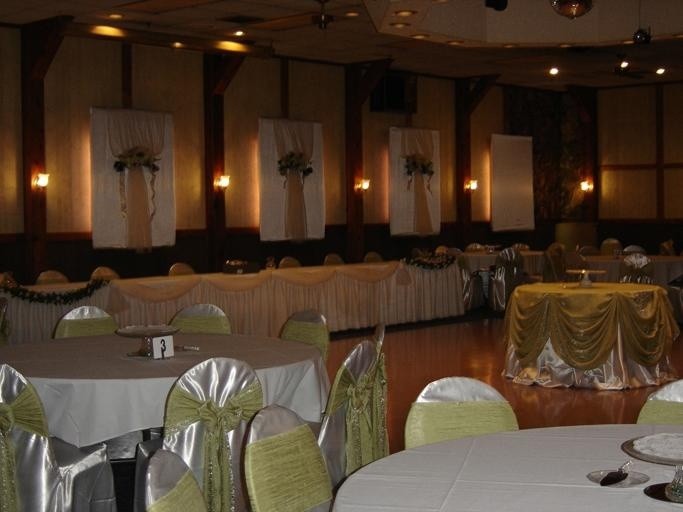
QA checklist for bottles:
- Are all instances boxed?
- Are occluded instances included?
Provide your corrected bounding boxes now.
[665,463,682,503]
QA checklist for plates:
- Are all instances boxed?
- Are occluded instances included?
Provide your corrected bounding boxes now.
[586,470,651,487]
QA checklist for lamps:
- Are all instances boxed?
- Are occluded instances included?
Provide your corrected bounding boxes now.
[466,180,478,191]
[35,173,51,188]
[358,179,370,192]
[580,180,594,192]
[214,175,230,189]
